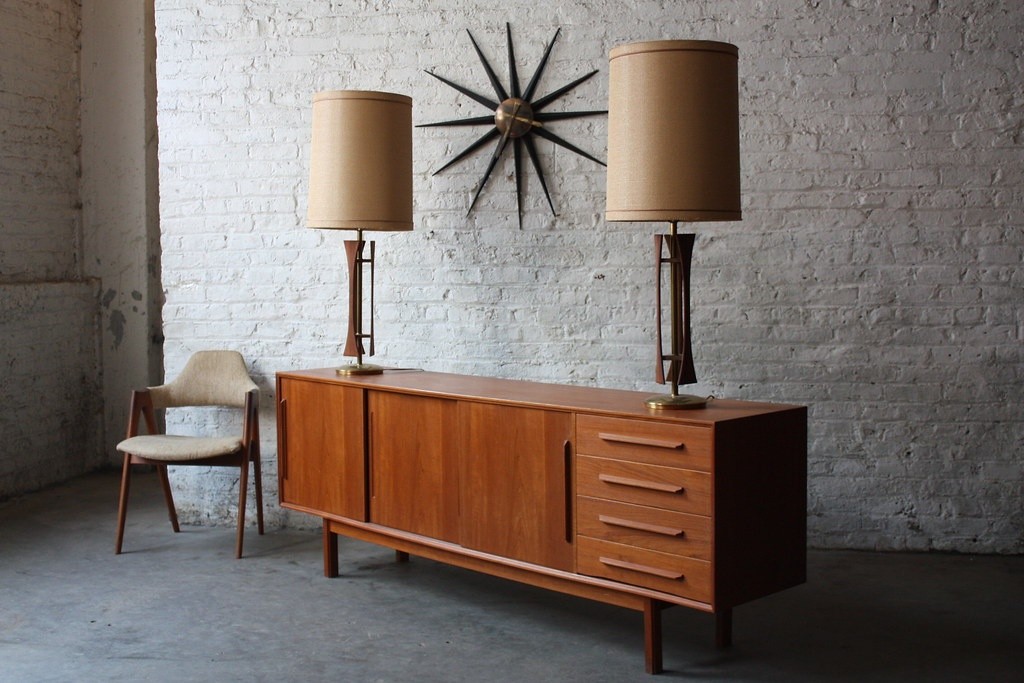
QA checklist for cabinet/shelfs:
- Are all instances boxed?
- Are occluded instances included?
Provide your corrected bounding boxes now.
[276,366,808,674]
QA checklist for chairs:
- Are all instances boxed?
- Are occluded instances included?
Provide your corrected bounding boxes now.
[115,350,264,559]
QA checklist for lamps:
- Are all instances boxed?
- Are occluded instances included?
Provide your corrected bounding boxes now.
[605,39,742,409]
[305,91,413,377]
